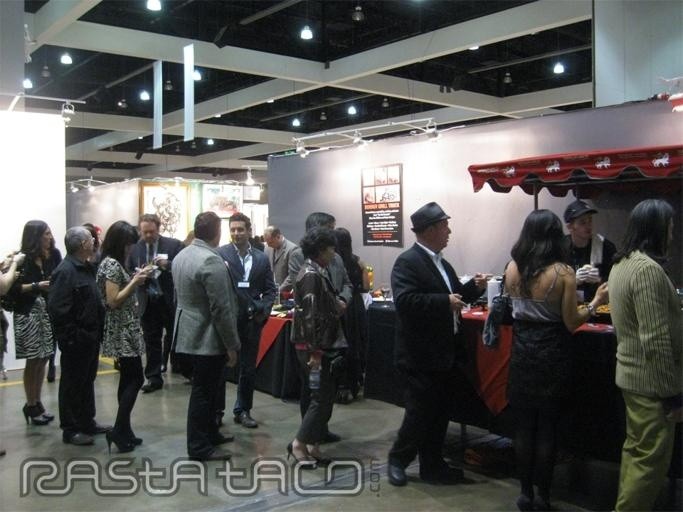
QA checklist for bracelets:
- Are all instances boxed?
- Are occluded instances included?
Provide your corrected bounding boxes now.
[587,303,596,317]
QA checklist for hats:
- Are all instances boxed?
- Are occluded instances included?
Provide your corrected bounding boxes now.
[564,200,597,221]
[410,203,450,231]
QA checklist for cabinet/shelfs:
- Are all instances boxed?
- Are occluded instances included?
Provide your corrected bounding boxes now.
[308,366,320,405]
[486,277,501,308]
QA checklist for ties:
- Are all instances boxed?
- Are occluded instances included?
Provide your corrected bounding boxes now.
[149,243,154,265]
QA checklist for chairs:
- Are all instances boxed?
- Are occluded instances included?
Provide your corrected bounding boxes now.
[64,432,94,445]
[94,423,113,434]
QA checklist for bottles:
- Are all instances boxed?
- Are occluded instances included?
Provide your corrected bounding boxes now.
[564,200,597,221]
[410,203,450,231]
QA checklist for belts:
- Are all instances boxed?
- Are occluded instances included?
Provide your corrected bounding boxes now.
[379,281,391,308]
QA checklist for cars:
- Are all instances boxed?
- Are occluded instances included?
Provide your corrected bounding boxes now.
[158,254,169,270]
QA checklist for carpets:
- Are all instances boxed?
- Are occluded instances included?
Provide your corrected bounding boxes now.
[149,243,154,265]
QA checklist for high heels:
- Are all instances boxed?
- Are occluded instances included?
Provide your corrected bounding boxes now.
[24,402,55,425]
[107,424,142,453]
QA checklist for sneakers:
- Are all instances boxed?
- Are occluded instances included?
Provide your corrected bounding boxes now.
[64,432,94,445]
[94,423,113,434]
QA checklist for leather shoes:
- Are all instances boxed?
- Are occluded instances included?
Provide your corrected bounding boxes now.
[586,268,601,284]
[142,364,558,511]
[575,267,589,286]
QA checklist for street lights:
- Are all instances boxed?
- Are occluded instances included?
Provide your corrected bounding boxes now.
[482,295,508,348]
[0,264,26,312]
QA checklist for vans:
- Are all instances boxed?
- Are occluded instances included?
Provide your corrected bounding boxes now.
[24,402,55,425]
[107,424,142,453]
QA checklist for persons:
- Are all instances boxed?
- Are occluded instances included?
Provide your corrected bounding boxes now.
[386,202,495,487]
[558,201,618,305]
[608,198,683,511]
[0,208,370,470]
[502,210,609,512]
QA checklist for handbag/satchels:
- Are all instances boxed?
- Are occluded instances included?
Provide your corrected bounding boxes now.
[587,303,596,317]
[0,264,26,312]
[482,295,508,348]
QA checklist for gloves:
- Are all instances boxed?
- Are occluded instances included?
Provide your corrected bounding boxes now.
[575,267,589,286]
[586,268,601,284]
